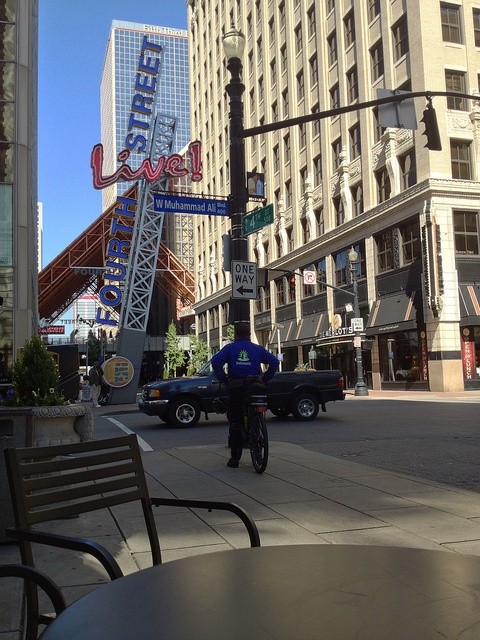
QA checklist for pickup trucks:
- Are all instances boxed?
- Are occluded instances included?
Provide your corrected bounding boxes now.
[138,357,345,427]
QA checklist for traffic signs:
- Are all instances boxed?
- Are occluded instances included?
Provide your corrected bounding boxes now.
[242,204,273,235]
[351,318,363,331]
[232,260,257,299]
[153,194,229,216]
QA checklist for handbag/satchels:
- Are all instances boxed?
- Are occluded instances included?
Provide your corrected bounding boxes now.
[212,383,229,415]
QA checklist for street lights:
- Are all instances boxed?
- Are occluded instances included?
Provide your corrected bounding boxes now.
[221,29,249,321]
[347,246,368,396]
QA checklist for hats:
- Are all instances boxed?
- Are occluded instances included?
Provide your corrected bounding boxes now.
[235,323,251,342]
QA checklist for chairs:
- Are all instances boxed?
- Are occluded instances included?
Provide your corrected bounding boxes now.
[4,434,260,640]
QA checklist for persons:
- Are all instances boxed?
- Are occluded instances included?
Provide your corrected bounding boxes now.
[89,361,104,408]
[212,323,278,467]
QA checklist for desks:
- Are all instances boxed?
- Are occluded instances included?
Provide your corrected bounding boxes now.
[39,544,480,640]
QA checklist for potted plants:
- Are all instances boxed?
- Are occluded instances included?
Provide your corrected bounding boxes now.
[0,332,93,444]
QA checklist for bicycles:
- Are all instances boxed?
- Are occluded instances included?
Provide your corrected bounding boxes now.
[244,374,269,474]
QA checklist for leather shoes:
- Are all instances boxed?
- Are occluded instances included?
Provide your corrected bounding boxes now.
[227,459,239,469]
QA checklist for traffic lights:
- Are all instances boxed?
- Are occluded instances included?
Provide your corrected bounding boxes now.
[290,276,295,294]
[420,108,442,151]
